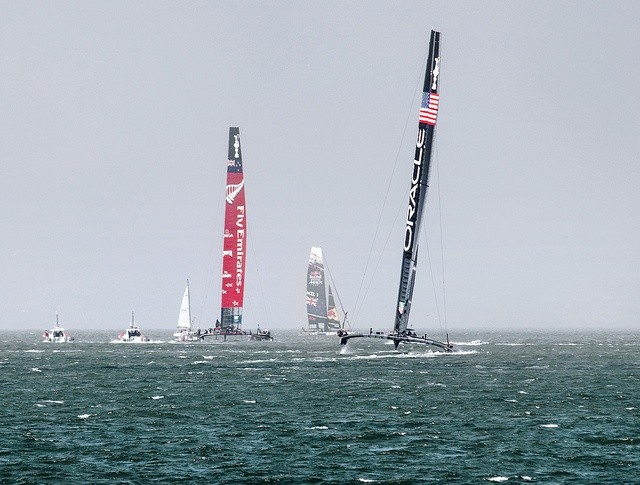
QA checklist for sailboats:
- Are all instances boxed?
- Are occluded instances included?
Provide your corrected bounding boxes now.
[299,247,355,335]
[181,127,273,341]
[173,279,195,337]
[337,29,452,355]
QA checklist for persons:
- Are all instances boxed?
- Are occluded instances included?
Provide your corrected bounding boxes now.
[257,324,261,335]
[215,319,221,328]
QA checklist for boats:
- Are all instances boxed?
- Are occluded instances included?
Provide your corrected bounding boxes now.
[118,312,150,342]
[43,314,75,343]
